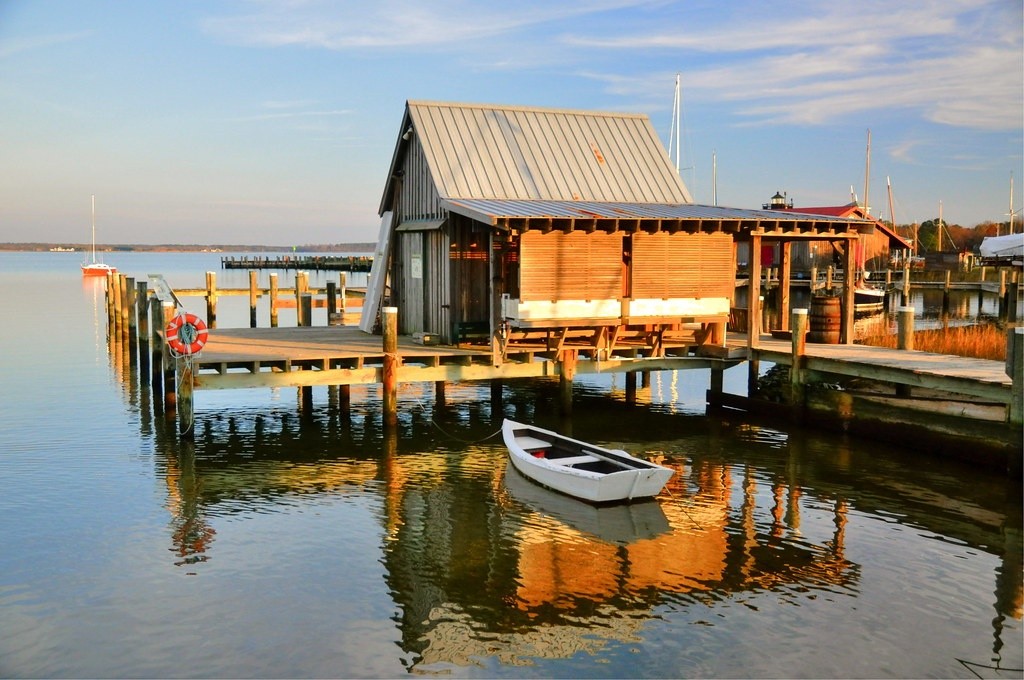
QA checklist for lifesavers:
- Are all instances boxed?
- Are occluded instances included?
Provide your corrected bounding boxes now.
[166,314,208,354]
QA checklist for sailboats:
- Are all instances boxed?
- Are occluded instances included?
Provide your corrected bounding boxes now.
[81,195,118,275]
[840,129,887,315]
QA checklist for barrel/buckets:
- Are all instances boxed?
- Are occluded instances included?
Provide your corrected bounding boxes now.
[809,295,841,344]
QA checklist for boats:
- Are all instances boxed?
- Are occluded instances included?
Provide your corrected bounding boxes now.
[501,417,675,505]
[506,456,675,547]
